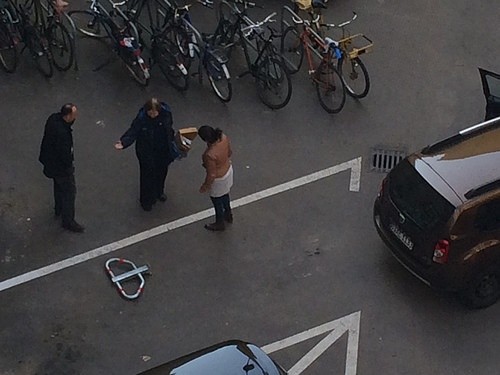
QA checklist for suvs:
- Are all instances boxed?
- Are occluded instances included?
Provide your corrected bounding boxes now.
[373,66,500,310]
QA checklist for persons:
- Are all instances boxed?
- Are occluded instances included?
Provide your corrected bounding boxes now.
[115,99,178,212]
[39,103,87,232]
[210,125,234,224]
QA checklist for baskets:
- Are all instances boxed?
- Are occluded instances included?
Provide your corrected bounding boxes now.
[338,34,375,58]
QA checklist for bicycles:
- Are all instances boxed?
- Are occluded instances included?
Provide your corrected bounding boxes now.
[0,0,374,114]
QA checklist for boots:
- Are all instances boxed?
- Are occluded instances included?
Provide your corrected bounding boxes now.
[223,210,233,223]
[205,217,225,230]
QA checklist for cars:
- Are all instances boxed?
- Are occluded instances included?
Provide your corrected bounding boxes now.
[136,340,290,375]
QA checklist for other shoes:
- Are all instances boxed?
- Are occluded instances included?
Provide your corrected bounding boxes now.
[143,203,152,211]
[157,192,166,202]
[56,209,63,216]
[62,219,84,232]
